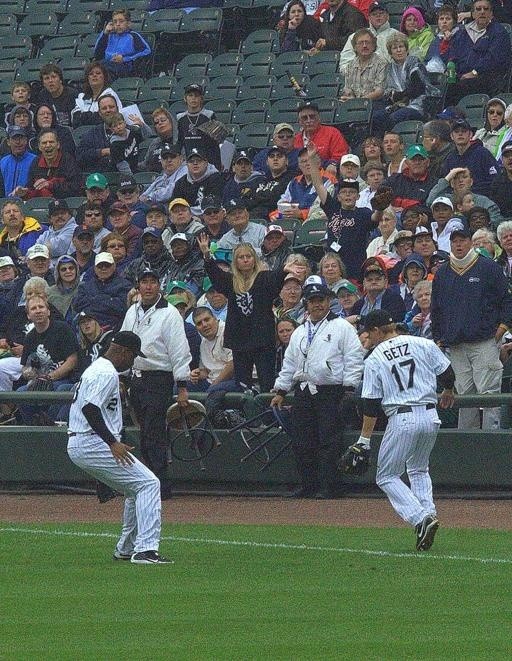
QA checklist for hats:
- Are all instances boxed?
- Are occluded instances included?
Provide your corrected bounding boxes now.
[437,106,465,119]
[369,2,387,13]
[396,322,409,332]
[297,101,317,112]
[113,332,147,359]
[186,83,205,95]
[357,310,393,336]
[486,97,508,110]
[77,312,95,323]
[501,141,512,154]
[0,121,470,306]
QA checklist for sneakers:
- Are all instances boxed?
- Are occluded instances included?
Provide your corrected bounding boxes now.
[131,551,175,565]
[113,548,136,558]
[416,515,440,550]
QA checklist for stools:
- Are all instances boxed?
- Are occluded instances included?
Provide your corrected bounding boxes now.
[229,405,295,473]
[164,400,221,472]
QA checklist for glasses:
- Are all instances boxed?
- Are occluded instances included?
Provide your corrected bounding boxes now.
[488,111,503,115]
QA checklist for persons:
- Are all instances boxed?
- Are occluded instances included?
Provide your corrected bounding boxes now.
[119,268,192,501]
[0,1,511,427]
[67,330,175,563]
[341,310,455,549]
[271,284,363,500]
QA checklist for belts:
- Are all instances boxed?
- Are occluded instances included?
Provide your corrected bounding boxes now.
[397,404,435,412]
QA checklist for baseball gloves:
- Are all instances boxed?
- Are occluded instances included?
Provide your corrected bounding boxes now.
[336,442,374,479]
[371,184,393,211]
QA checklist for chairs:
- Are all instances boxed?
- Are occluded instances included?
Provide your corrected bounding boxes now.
[0,0,512,245]
